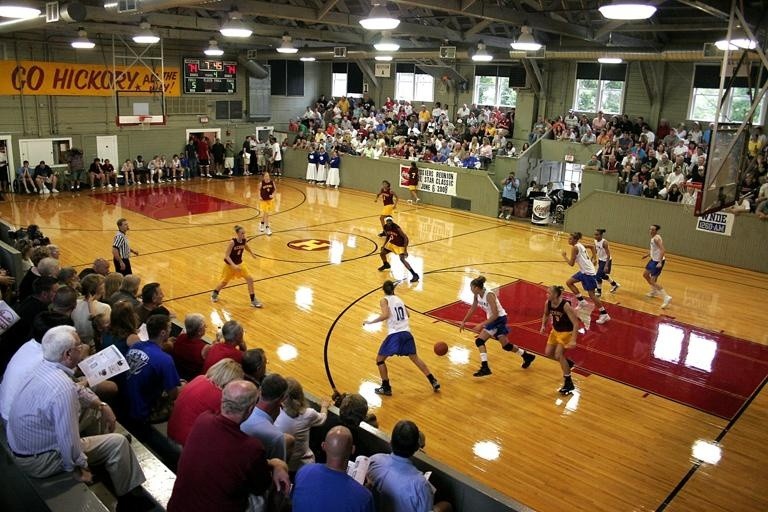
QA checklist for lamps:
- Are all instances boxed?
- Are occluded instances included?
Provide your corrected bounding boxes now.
[358,1,401,30]
[597,1,657,20]
[70,24,96,49]
[375,56,393,61]
[276,31,298,54]
[714,2,759,51]
[219,5,254,38]
[597,31,623,64]
[300,57,316,62]
[509,19,541,50]
[1,2,42,19]
[132,19,160,43]
[203,35,224,56]
[373,31,401,51]
[472,39,493,63]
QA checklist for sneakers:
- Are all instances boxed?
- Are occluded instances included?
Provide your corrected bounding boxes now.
[258,220,271,235]
[407,271,421,284]
[644,285,672,310]
[249,298,263,309]
[22,169,282,194]
[404,195,422,206]
[472,352,578,395]
[373,382,393,396]
[377,262,391,270]
[427,378,441,392]
[498,211,510,221]
[211,289,220,304]
[574,283,621,324]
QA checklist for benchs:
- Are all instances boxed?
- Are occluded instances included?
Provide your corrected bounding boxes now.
[2,440,109,512]
[85,420,178,511]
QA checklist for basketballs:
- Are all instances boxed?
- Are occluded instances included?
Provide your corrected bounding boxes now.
[433,341,448,357]
